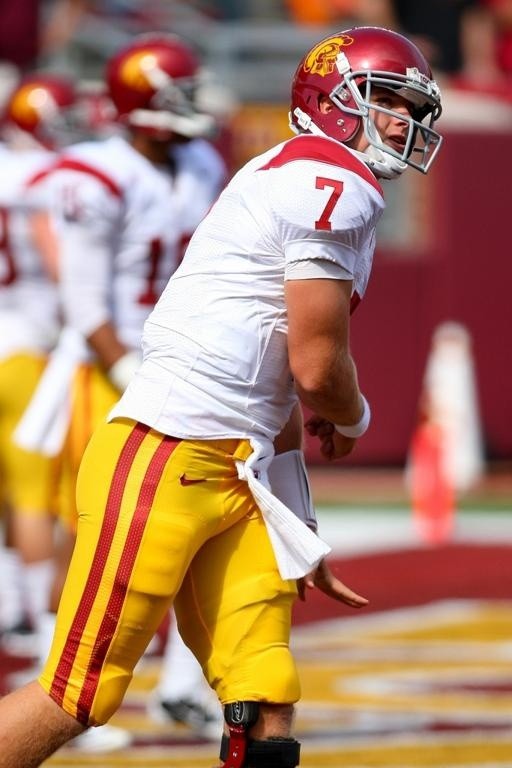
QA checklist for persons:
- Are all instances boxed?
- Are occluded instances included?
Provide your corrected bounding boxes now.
[283,1,512,132]
[0,28,443,767]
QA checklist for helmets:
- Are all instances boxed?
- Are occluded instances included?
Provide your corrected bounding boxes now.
[287,22,448,145]
[1,69,78,154]
[100,27,209,119]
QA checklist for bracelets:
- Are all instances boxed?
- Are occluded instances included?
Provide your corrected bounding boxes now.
[269,451,315,530]
[333,390,373,440]
